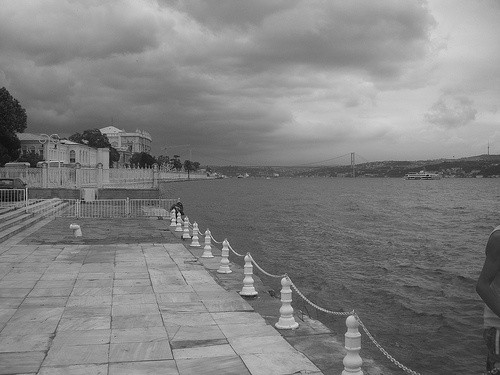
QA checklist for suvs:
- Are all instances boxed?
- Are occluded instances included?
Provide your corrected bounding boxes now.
[0,178,29,201]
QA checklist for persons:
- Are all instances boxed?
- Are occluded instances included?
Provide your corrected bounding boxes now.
[475,222,499,374]
